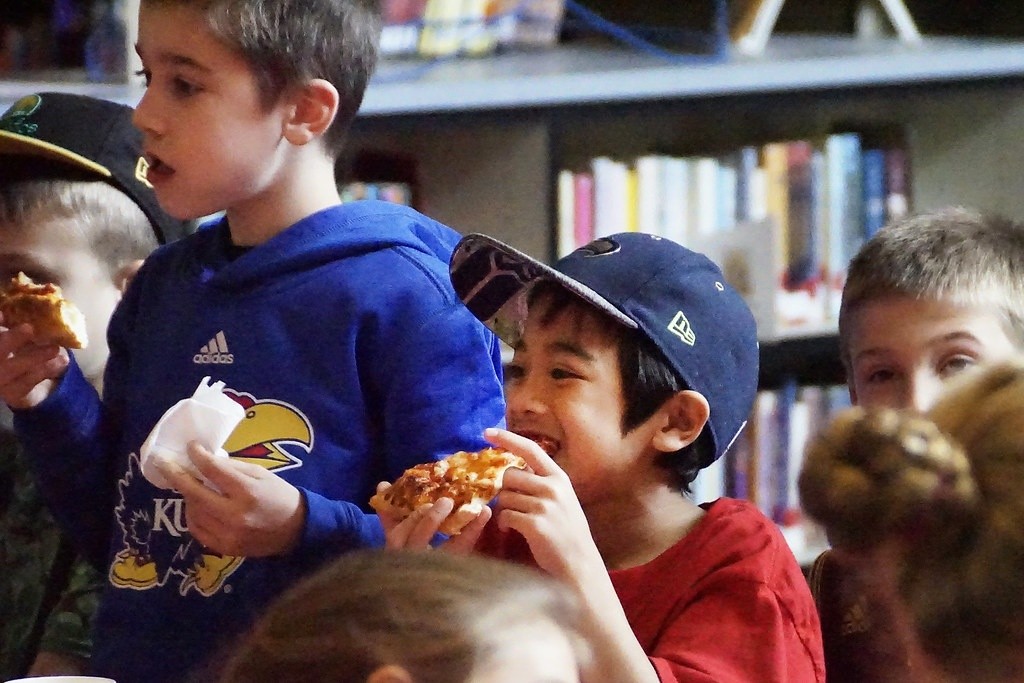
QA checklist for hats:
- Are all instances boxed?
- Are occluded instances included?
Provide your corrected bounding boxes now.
[450,232,759,461]
[1,92,182,245]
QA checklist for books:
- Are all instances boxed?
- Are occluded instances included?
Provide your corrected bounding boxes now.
[380,1,920,62]
[549,133,913,338]
[680,378,857,566]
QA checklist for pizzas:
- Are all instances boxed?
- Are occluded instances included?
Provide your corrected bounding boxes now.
[370,438,558,535]
[0,271,90,349]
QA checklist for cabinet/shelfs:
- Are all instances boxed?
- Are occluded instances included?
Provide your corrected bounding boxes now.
[0,0,1024,563]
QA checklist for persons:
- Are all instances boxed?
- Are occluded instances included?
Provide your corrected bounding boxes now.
[217,544,591,683]
[794,361,1024,683]
[808,204,1024,683]
[0,90,196,683]
[1,1,504,683]
[375,231,827,683]
